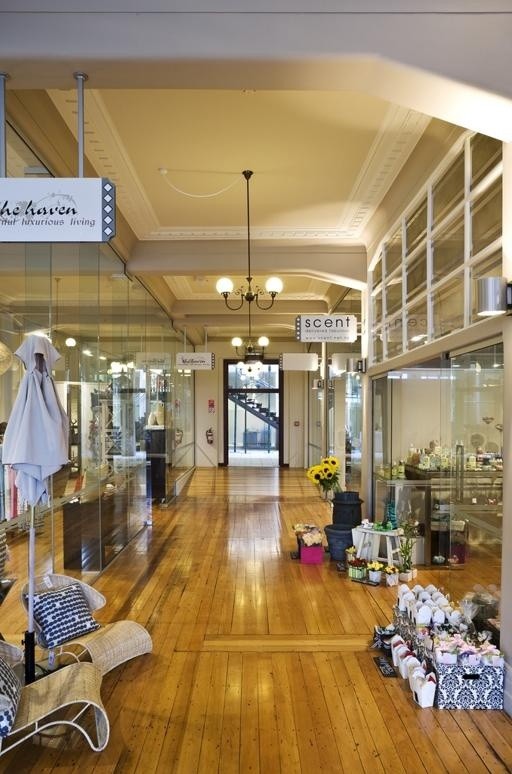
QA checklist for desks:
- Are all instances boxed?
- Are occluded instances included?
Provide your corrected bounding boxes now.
[397,461,502,523]
[62,487,128,570]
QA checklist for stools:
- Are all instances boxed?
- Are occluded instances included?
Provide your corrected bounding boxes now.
[355,526,403,569]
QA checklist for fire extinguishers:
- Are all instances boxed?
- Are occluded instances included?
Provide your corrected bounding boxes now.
[206,427,213,444]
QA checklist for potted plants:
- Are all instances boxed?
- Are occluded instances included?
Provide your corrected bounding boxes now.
[399,538,417,581]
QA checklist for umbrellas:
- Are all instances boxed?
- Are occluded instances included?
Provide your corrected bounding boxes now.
[1,335,71,686]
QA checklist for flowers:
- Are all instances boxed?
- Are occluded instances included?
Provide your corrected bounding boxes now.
[345,546,400,574]
[305,456,341,502]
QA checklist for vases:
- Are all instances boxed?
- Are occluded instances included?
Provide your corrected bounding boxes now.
[348,566,398,587]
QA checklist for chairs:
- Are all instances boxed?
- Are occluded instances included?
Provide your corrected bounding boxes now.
[0,572,154,757]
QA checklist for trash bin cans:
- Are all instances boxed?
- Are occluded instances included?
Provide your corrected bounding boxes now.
[450,520,470,570]
[431,521,450,566]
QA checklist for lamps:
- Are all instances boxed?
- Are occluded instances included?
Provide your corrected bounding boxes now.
[346,358,364,372]
[216,169,283,381]
[311,379,324,389]
[473,277,512,318]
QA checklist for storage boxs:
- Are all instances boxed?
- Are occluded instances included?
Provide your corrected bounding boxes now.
[389,605,503,711]
